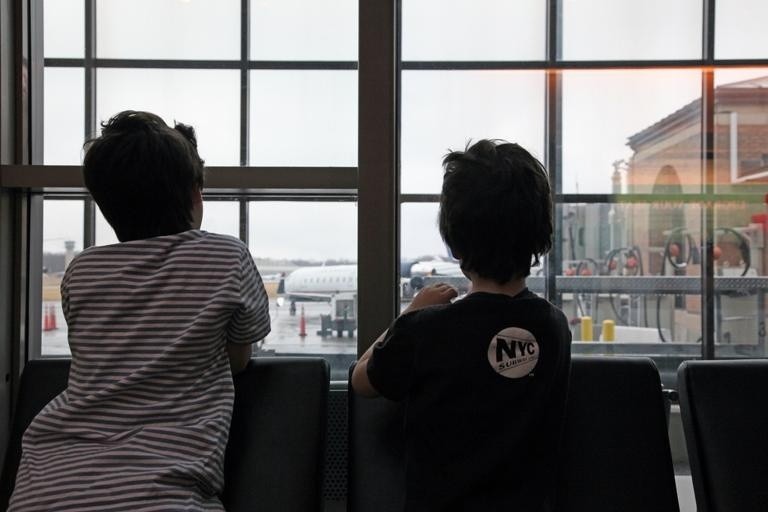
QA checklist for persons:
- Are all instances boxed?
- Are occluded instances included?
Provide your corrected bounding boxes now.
[349,138,573,510]
[3,109,273,512]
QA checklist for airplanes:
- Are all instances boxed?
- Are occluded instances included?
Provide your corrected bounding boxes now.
[277,261,458,316]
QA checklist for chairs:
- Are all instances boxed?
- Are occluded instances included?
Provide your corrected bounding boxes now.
[676,358,767,511]
[344,359,679,511]
[0,357,330,512]
